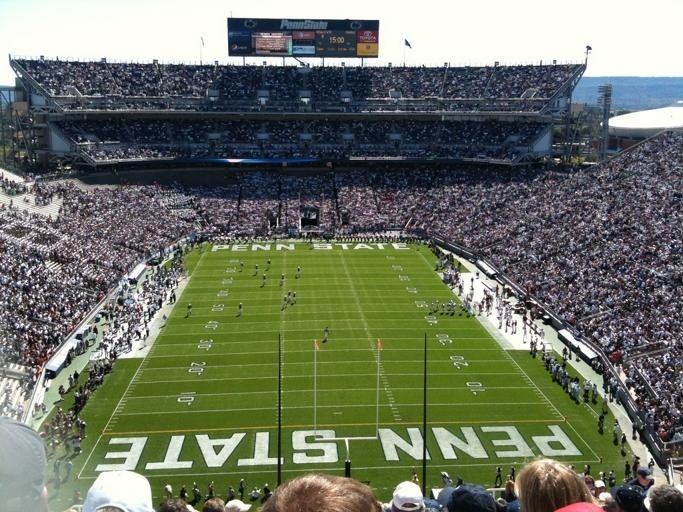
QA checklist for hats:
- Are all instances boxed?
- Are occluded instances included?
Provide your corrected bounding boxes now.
[0,418,46,510]
[638,467,654,480]
[594,480,606,488]
[81,470,156,512]
[225,499,252,512]
[392,480,424,511]
